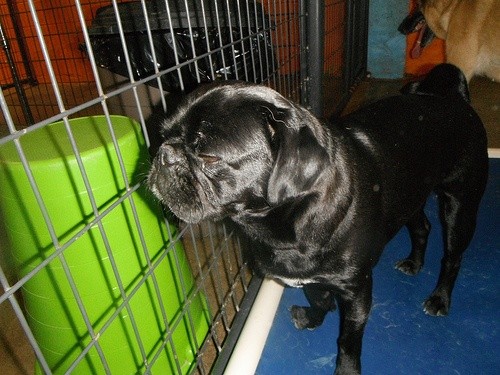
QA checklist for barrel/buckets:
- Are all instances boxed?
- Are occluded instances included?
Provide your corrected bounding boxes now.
[0,113,213,375]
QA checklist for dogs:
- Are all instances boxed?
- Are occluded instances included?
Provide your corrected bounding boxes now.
[139,63,489,375]
[397,0,500,89]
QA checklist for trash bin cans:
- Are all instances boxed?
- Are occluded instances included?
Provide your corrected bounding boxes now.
[79,0,287,243]
